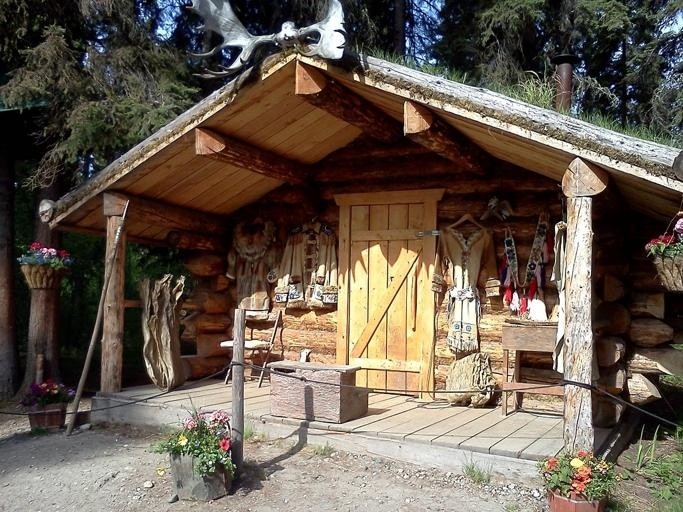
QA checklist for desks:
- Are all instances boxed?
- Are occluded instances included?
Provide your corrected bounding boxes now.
[502,323,565,416]
[267,359,372,423]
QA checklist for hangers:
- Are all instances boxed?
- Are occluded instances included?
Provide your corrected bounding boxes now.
[449,207,484,229]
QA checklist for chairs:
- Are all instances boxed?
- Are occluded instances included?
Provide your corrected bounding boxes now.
[309,215,320,234]
[220,310,282,388]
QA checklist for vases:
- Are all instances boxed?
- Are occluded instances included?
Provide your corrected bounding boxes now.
[546,486,607,512]
[169,451,231,502]
[27,402,64,434]
[654,254,683,291]
[21,264,64,289]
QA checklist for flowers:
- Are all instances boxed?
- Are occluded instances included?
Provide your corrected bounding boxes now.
[644,234,683,257]
[19,378,75,410]
[18,242,74,269]
[537,449,619,503]
[148,409,238,480]
